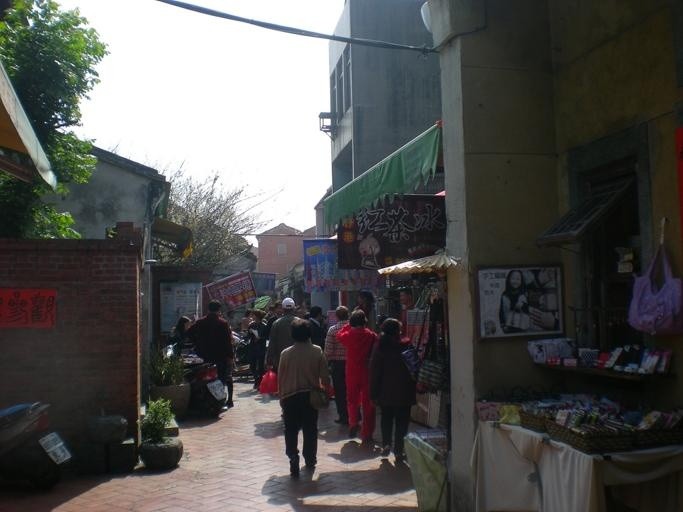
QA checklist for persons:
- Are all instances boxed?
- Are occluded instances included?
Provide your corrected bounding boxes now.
[265,298,311,372]
[170,300,234,407]
[336,310,376,444]
[324,306,362,424]
[295,306,325,352]
[368,318,419,462]
[277,319,331,473]
[241,302,282,393]
[500,270,529,333]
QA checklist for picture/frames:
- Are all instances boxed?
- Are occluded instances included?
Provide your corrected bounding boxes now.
[473,261,566,343]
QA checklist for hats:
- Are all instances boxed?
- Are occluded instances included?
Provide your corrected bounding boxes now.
[282,297,295,309]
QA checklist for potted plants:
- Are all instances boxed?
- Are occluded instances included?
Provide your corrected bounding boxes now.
[141,348,191,418]
[137,395,183,474]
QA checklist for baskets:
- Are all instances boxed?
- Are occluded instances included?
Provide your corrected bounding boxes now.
[519,408,682,455]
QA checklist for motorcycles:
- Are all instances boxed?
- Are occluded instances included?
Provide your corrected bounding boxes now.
[230,329,253,381]
[159,342,227,418]
[0,398,74,495]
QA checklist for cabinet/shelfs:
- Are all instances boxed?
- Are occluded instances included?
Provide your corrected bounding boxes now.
[582,267,643,353]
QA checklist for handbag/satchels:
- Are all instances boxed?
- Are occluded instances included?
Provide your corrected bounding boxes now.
[400,348,447,391]
[310,387,329,409]
[626,276,683,337]
[506,310,530,330]
[528,338,576,365]
